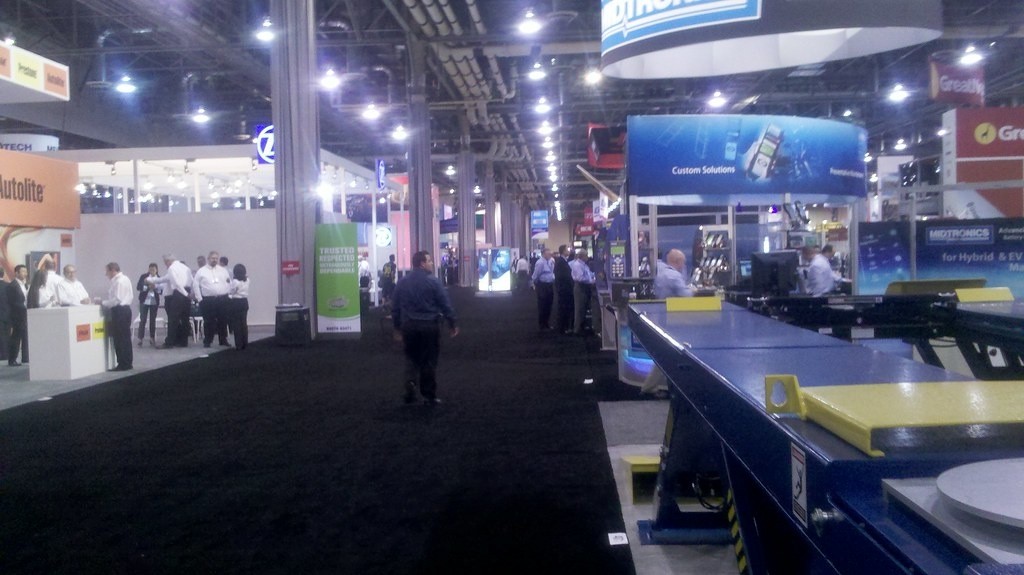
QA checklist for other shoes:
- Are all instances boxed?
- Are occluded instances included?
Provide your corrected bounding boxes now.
[126,364,133,370]
[108,365,128,371]
[156,344,173,348]
[424,397,444,406]
[150,341,155,345]
[204,343,210,347]
[219,340,231,346]
[176,344,188,347]
[138,342,142,345]
[405,382,417,395]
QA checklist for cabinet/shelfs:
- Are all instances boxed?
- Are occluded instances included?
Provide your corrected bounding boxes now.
[702,225,734,286]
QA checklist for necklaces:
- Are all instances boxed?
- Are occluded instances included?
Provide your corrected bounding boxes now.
[136,263,162,347]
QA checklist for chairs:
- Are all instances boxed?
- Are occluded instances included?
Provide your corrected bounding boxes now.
[132,312,204,343]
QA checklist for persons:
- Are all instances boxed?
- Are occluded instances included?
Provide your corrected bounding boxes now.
[7,265,29,365]
[637,248,700,401]
[226,264,250,349]
[145,274,171,315]
[27,269,56,308]
[43,259,64,298]
[55,264,91,306]
[391,250,461,404]
[794,245,854,296]
[180,255,233,339]
[194,251,231,347]
[0,268,10,359]
[102,262,134,371]
[357,243,668,336]
[158,253,194,348]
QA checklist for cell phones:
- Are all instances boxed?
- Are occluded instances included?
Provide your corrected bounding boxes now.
[747,122,784,182]
[609,240,627,279]
[724,118,743,162]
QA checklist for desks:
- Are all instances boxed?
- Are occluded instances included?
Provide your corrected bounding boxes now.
[611,277,1024,575]
[26,304,119,379]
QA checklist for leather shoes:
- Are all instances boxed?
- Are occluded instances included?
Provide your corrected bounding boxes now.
[22,359,29,362]
[9,360,21,366]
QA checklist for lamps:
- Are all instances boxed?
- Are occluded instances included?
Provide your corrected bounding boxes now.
[184,159,196,173]
[104,161,116,176]
[525,45,550,78]
[251,157,257,171]
[114,53,139,92]
[193,70,212,121]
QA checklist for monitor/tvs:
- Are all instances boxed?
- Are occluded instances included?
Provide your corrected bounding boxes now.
[751,250,797,297]
